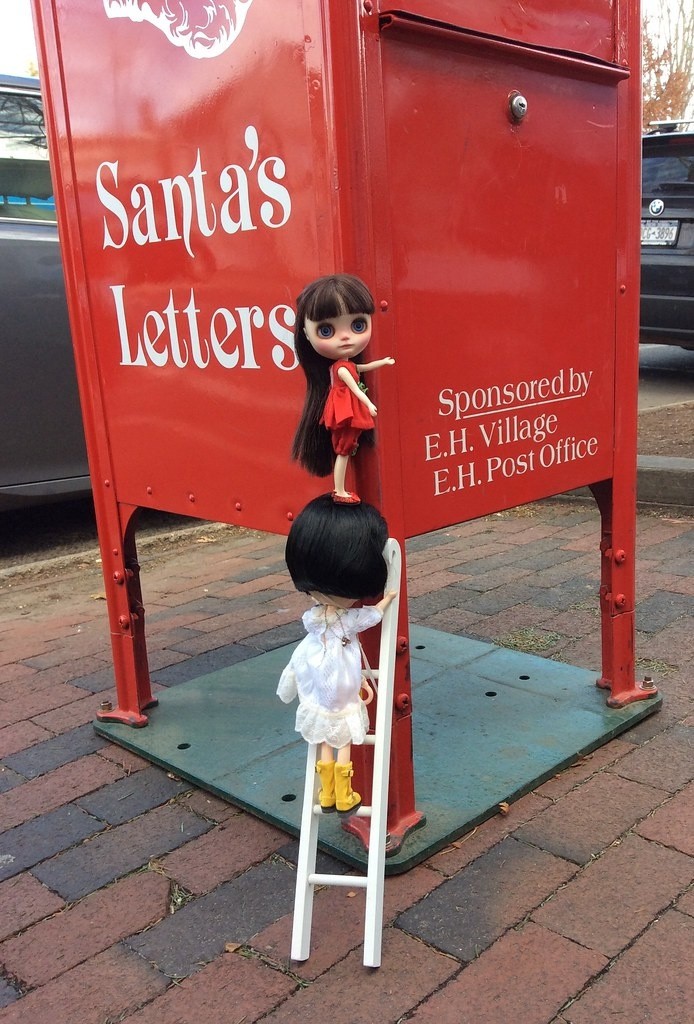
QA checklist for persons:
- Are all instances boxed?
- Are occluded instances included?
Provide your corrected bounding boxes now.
[275,489,397,819]
[290,272,395,505]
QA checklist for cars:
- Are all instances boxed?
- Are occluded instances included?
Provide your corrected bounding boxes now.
[642,120,694,358]
[0,73,95,498]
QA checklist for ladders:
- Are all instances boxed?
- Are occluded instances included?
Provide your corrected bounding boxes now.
[289,537,408,966]
